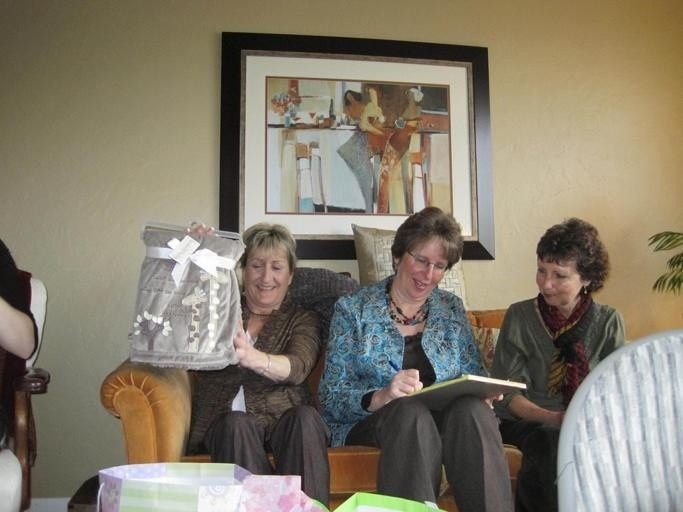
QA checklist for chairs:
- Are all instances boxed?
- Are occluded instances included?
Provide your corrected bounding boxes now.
[2,269,49,511]
[557,329,682,511]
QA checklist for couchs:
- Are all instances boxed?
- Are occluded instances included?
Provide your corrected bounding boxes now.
[99,307,524,512]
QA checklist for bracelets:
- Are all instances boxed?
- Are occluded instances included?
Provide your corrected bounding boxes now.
[260,354,271,377]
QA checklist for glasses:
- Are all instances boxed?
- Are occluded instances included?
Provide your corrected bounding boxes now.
[407,251,447,271]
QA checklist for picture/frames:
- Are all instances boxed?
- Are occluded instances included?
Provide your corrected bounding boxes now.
[217,29,498,262]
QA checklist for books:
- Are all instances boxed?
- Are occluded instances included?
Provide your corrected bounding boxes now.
[385,373,527,411]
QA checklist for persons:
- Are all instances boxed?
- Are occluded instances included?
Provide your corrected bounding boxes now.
[0,240,38,512]
[490,217,626,512]
[318,207,515,512]
[185,222,331,510]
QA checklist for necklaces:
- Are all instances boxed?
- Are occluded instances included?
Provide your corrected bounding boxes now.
[386,283,429,326]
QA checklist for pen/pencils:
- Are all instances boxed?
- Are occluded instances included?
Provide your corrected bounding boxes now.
[389,359,401,372]
